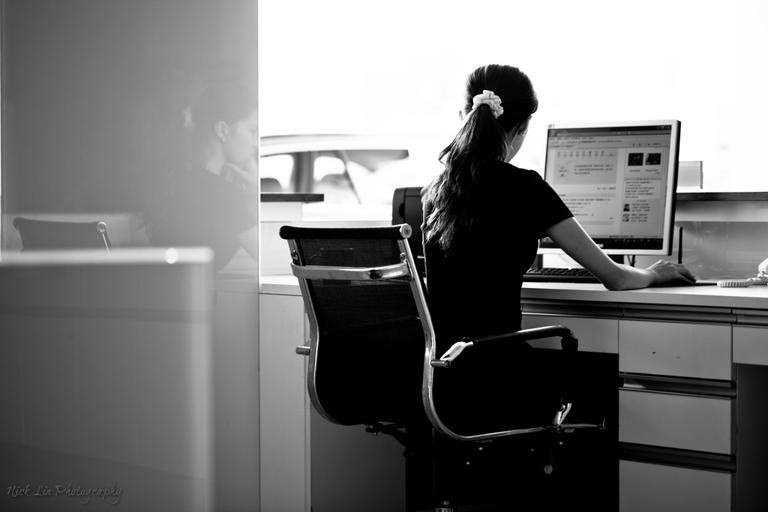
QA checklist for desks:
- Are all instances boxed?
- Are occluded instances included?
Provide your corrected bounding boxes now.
[258,275,768,511]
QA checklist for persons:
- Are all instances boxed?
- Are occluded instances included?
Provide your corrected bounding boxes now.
[420,64,696,427]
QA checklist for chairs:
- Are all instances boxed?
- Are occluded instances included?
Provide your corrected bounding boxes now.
[278,224,607,510]
[13,216,111,254]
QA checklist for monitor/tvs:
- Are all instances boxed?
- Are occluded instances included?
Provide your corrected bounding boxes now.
[536,119,682,264]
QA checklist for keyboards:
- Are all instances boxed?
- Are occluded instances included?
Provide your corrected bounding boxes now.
[523,267,601,283]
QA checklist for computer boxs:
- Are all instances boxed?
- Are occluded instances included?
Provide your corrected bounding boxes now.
[392,187,426,277]
